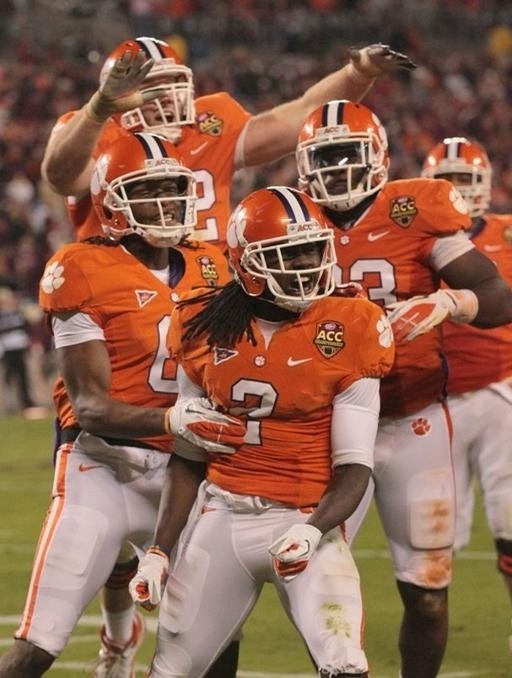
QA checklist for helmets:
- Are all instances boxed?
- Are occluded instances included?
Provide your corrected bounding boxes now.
[90,132,197,248]
[296,100,391,212]
[98,38,197,134]
[422,137,493,217]
[225,185,338,312]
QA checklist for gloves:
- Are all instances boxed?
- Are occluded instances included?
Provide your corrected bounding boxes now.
[268,524,323,583]
[129,546,169,610]
[384,288,460,346]
[348,44,418,85]
[164,397,247,454]
[85,50,165,122]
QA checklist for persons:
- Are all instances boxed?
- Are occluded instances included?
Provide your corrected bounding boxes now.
[293,100,510,675]
[0,0,511,415]
[1,130,248,678]
[419,135,511,587]
[126,185,395,678]
[42,37,415,677]
